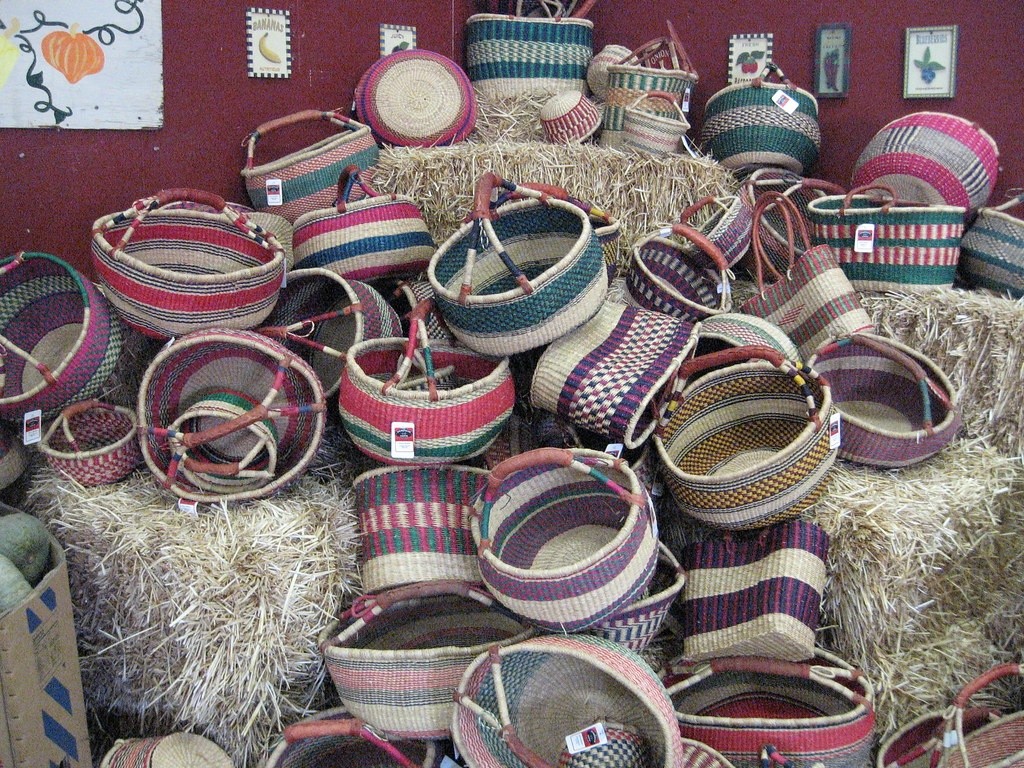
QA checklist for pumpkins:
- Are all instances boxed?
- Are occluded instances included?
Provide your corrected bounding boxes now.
[0,510,49,615]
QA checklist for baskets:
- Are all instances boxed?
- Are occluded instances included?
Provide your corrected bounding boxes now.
[0,0,1024,768]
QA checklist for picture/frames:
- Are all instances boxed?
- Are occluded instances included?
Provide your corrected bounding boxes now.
[904,23,958,98]
[727,34,775,81]
[379,23,417,56]
[816,26,851,99]
[245,6,292,78]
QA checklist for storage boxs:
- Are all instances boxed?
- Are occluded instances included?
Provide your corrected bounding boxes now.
[0,502,96,768]
